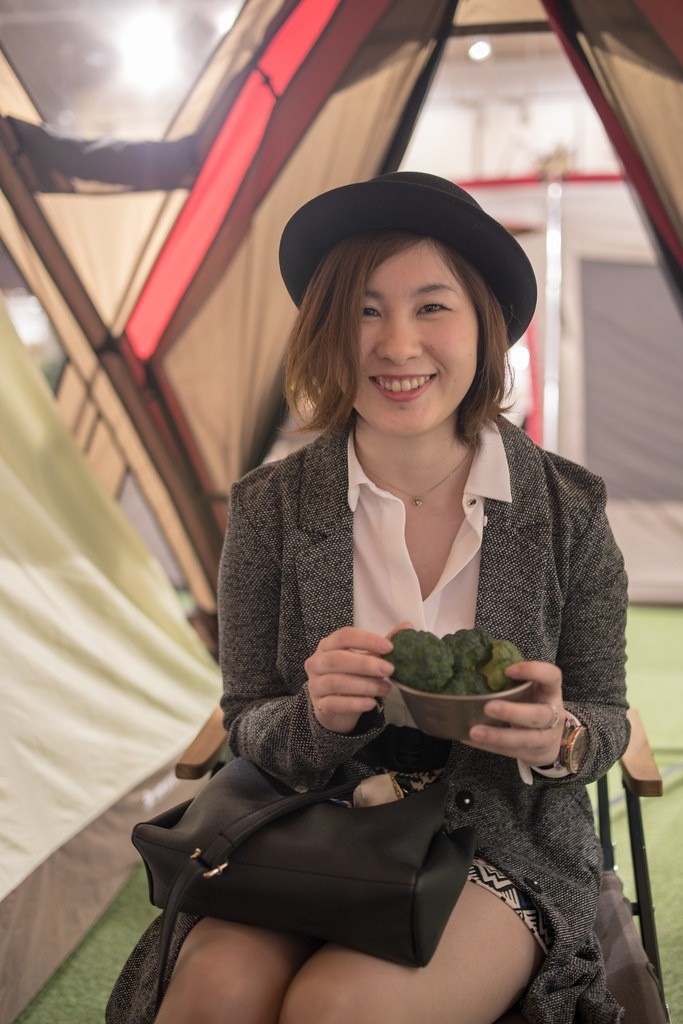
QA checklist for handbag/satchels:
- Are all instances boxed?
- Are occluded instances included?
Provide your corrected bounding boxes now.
[139,759,476,967]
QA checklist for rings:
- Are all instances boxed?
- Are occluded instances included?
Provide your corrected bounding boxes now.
[536,698,559,729]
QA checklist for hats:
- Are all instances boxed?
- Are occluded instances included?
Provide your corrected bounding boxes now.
[279,172,537,351]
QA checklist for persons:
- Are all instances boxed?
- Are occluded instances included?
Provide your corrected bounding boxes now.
[103,162,631,1024]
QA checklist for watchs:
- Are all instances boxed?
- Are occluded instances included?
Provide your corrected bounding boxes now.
[515,710,588,786]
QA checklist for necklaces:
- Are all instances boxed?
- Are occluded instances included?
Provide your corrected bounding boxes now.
[356,441,474,507]
